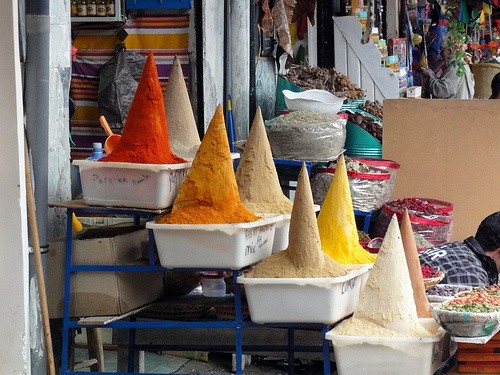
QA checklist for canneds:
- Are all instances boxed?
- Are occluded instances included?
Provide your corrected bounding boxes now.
[77,1,87,17]
[97,1,107,17]
[87,1,97,16]
[71,1,77,17]
[107,0,115,17]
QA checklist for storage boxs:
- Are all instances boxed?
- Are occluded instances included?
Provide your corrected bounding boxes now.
[46,148,461,375]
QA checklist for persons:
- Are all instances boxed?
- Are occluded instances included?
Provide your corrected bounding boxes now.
[421,40,476,99]
[490,72,500,99]
[419,210,500,288]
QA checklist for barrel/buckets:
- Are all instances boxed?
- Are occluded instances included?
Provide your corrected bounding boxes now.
[273,74,382,159]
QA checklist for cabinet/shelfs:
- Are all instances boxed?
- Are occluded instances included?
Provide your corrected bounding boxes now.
[46,198,335,375]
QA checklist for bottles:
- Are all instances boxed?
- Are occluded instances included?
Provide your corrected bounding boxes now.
[203,273,226,298]
[92,142,103,159]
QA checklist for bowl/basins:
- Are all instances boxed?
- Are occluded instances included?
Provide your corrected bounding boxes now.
[282,88,348,114]
[426,272,500,337]
